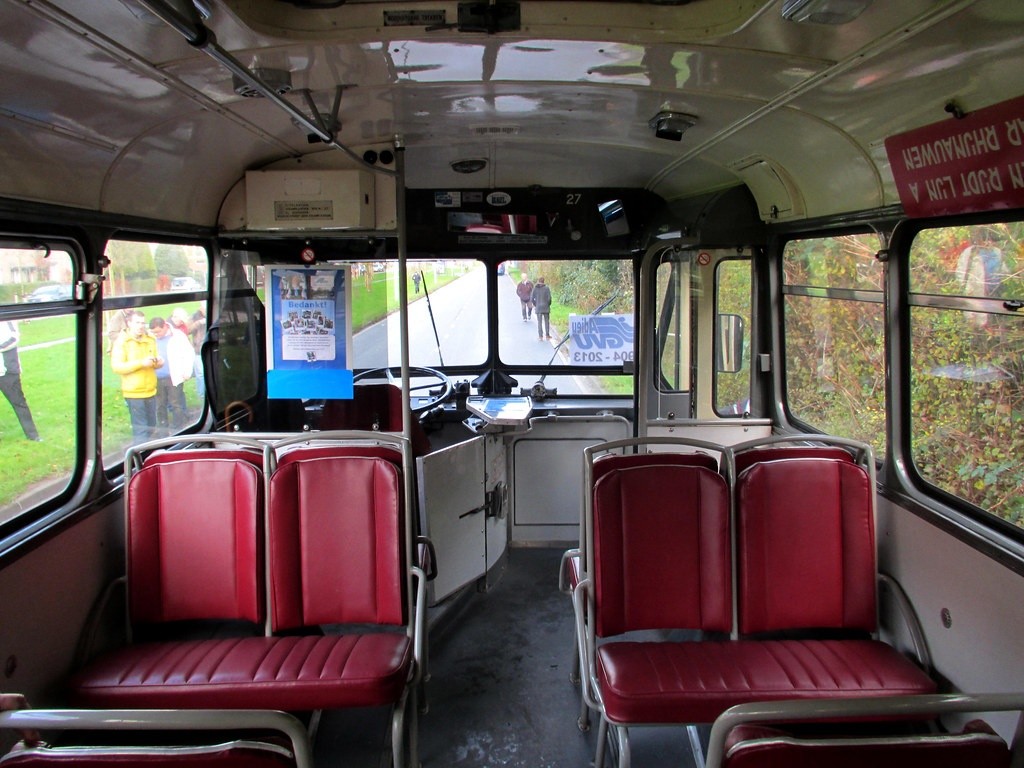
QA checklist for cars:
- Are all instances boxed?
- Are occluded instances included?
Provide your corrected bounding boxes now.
[22,284,73,302]
[170,277,202,292]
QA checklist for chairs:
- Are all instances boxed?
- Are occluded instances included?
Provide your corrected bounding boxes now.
[321,384,432,456]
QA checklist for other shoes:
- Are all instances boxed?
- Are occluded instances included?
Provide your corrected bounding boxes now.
[34,435,44,442]
[523,318,527,323]
[538,336,543,340]
[545,335,552,339]
[527,315,532,320]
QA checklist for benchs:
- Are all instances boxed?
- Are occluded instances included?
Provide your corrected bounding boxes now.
[556,429,1024,768]
[0,430,428,768]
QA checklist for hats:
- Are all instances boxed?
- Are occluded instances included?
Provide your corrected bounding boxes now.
[171,306,188,325]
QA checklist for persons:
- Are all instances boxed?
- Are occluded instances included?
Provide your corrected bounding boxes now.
[286,275,303,299]
[956,228,1008,326]
[531,277,552,339]
[107,309,207,445]
[0,321,43,442]
[412,272,421,293]
[516,273,534,321]
